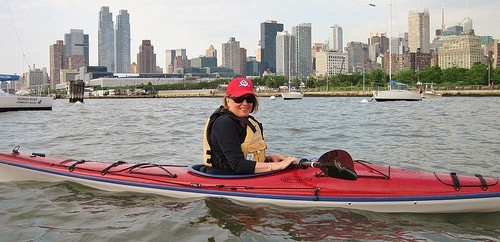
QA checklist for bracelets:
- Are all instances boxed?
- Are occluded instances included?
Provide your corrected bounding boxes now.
[269,162,273,172]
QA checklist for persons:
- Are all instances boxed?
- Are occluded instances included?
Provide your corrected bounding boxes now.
[202,77,299,176]
[419,85,423,94]
[489,81,494,90]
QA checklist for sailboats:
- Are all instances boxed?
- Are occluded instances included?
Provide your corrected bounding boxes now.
[372,0,422,102]
[282,34,303,100]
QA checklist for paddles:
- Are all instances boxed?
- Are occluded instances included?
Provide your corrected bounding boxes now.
[264,149,354,178]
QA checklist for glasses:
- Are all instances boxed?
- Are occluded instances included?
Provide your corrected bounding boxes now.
[227,96,254,103]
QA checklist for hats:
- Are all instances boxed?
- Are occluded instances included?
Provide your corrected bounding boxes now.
[226,78,257,97]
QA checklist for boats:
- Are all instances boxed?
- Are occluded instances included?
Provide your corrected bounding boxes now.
[0,152,500,213]
